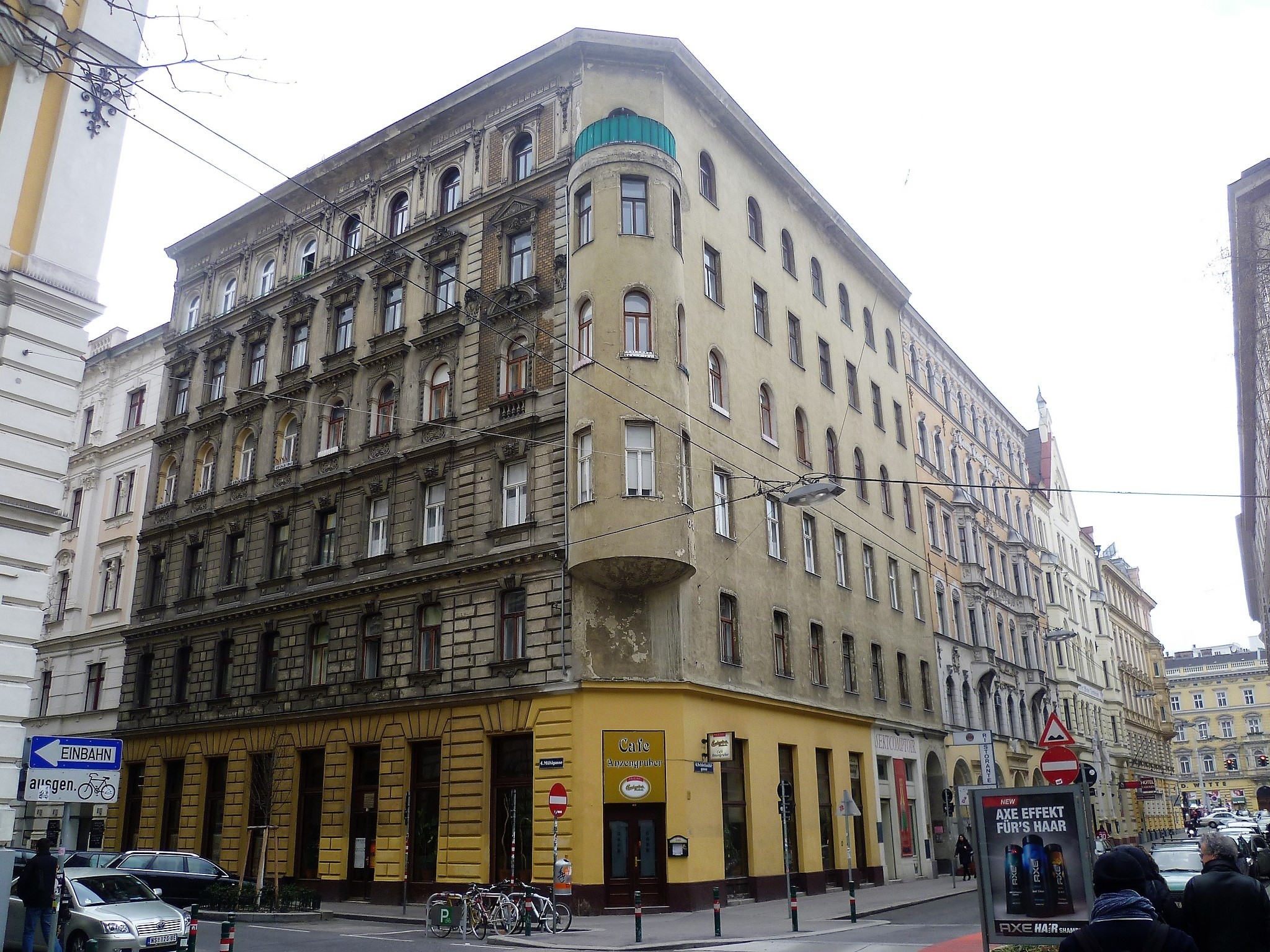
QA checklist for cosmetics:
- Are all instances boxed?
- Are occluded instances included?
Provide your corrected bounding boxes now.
[1004,835,1075,917]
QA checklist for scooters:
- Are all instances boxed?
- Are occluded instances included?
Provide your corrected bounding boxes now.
[1188,822,1199,839]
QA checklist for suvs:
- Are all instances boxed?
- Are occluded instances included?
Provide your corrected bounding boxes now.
[105,849,285,913]
[3,846,123,870]
[1148,836,1204,910]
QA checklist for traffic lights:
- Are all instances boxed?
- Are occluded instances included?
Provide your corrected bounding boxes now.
[1265,756,1270,765]
[1260,755,1266,766]
[1231,759,1236,769]
[1257,756,1261,765]
[1226,759,1235,771]
[1223,760,1228,768]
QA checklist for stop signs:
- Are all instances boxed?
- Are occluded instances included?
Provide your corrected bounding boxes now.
[550,784,571,820]
[1038,745,1079,785]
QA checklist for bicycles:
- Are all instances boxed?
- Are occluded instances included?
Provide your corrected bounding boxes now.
[430,876,572,939]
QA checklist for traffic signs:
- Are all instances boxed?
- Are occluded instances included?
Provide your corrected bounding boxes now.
[30,735,125,774]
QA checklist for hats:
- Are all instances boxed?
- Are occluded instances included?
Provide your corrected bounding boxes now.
[1093,851,1146,897]
[1109,844,1156,878]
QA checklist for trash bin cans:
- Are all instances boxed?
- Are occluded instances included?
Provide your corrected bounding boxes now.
[553,858,571,895]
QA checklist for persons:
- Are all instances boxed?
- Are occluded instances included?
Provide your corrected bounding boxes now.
[1182,834,1270,952]
[1060,850,1196,952]
[955,834,973,880]
[1187,823,1197,837]
[1192,810,1197,817]
[1236,810,1239,816]
[16,838,62,952]
[1117,845,1182,930]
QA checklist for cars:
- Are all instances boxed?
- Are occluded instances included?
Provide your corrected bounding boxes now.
[1193,803,1270,887]
[4,867,190,952]
[1199,812,1254,828]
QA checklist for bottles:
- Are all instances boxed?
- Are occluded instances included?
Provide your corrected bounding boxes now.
[1004,844,1027,914]
[1022,835,1050,918]
[1044,843,1074,916]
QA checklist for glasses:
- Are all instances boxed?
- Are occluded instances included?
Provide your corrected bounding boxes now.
[1199,853,1214,859]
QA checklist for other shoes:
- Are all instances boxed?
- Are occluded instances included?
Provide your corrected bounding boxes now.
[968,876,971,881]
[963,878,966,881]
